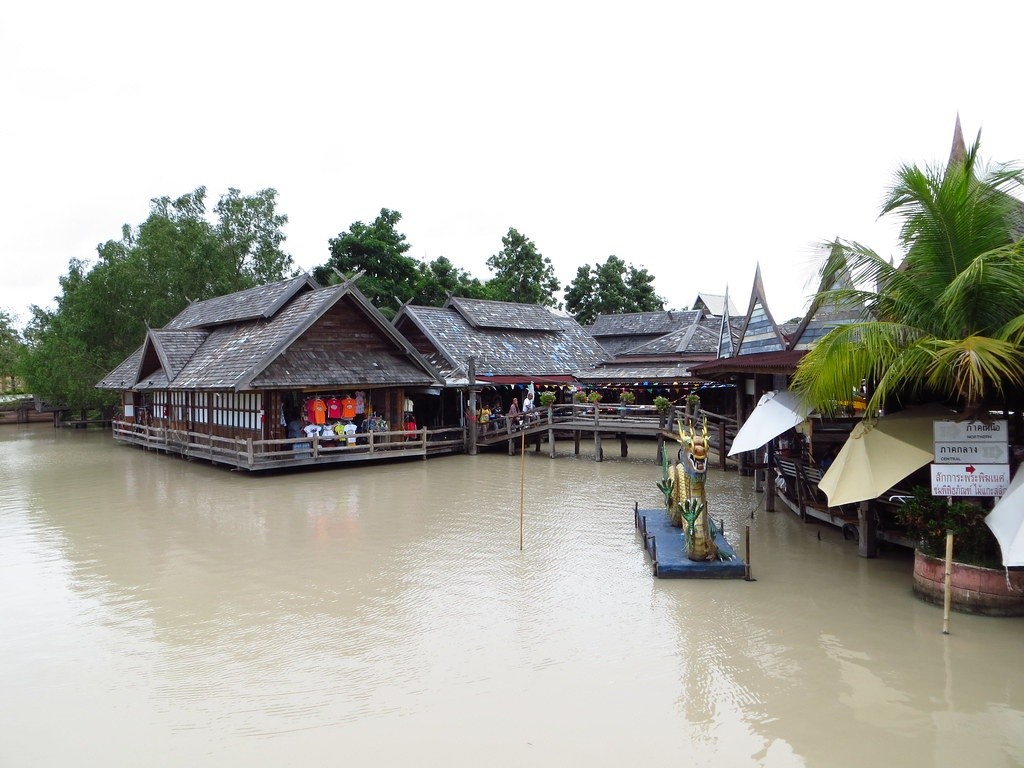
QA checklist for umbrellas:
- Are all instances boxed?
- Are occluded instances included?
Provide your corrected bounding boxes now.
[726,388,823,472]
[982,456,1024,570]
[817,413,958,506]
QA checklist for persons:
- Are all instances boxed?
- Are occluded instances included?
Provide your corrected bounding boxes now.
[521,393,536,428]
[465,400,506,440]
[508,397,522,432]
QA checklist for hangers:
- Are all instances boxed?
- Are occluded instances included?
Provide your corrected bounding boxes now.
[302,394,361,431]
